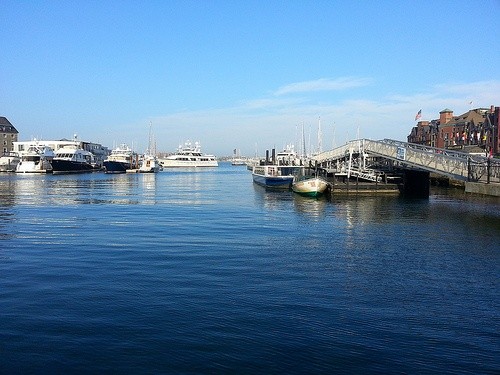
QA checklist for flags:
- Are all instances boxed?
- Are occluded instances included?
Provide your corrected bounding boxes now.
[415,110,422,121]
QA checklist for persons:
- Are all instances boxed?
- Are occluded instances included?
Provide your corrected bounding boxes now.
[436,150,440,154]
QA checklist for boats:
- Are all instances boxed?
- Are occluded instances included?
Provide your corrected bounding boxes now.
[0,139,53,173]
[292,160,333,196]
[52,142,100,176]
[159,137,218,167]
[251,144,300,187]
[231,149,261,171]
[103,121,165,174]
